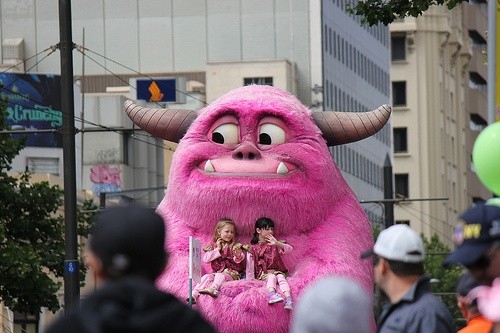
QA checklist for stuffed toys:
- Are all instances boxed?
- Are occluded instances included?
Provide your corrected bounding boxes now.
[124,85,391,333]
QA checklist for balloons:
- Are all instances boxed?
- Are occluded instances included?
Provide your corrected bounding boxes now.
[472,122,500,206]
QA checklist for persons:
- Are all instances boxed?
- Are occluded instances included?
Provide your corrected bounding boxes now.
[290,278,373,333]
[187,220,246,304]
[244,218,293,310]
[360,205,500,333]
[44,205,219,333]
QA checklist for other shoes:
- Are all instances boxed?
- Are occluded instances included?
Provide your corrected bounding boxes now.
[186,297,197,304]
[284,302,293,310]
[199,288,217,298]
[268,296,283,304]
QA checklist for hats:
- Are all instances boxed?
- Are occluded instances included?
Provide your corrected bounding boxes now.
[360,223,425,265]
[440,206,500,265]
[89,204,166,280]
